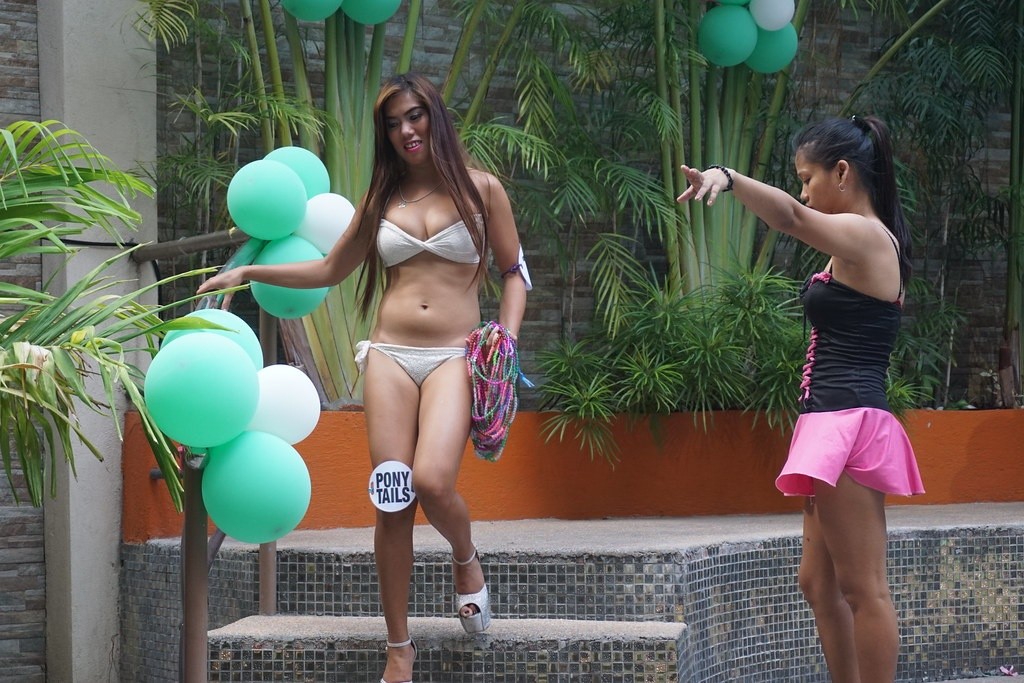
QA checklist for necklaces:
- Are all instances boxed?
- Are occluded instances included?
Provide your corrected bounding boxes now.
[398,179,444,203]
[465,321,535,462]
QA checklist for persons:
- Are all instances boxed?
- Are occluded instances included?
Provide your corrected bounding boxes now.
[677,115,927,683]
[197,74,527,683]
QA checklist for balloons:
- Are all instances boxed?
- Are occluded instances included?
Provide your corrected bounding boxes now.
[698,0,798,74]
[280,0,402,25]
[227,146,356,319]
[144,309,321,543]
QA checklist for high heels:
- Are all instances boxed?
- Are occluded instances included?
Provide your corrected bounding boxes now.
[452,547,491,634]
[380,635,417,683]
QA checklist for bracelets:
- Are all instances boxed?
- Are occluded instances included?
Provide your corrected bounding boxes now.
[705,164,733,192]
